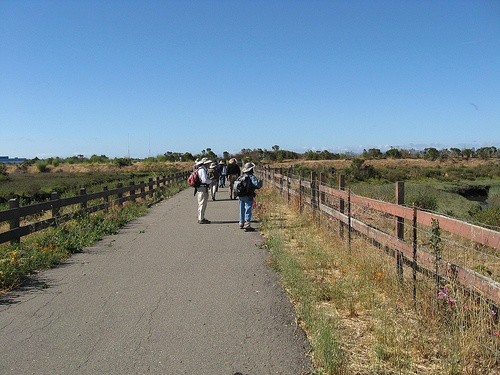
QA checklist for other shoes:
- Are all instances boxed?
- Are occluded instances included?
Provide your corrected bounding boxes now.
[244,223,255,232]
[239,222,245,229]
[199,221,211,224]
[197,219,208,223]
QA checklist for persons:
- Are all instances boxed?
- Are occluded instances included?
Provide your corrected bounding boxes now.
[226,158,240,199]
[234,163,262,231]
[188,159,227,224]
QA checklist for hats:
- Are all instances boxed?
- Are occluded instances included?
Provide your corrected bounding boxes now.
[219,160,225,165]
[203,158,212,164]
[195,159,206,166]
[242,162,255,173]
[211,163,217,168]
[229,158,238,165]
[195,159,200,162]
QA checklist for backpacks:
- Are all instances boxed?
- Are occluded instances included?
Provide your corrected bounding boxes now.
[234,174,255,197]
[188,167,205,187]
